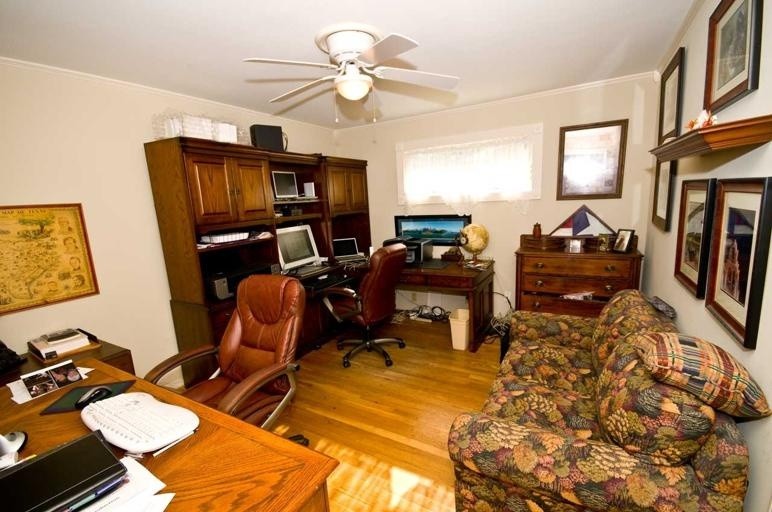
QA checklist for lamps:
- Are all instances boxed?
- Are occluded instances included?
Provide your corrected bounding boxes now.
[334,76,372,102]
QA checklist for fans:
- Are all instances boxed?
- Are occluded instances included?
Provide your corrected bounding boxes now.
[245,23,461,104]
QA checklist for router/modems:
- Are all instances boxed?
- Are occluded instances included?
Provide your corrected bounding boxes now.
[303,182,315,196]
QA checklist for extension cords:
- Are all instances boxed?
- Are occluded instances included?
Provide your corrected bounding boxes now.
[415,315,433,322]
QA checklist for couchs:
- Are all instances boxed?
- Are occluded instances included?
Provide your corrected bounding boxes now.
[448,309,750,512]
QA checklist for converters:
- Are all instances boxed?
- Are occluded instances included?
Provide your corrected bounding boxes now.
[421,312,432,318]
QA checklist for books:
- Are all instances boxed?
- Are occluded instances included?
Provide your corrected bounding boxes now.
[0,429,128,512]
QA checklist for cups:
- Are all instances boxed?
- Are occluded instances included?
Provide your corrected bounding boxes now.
[303,181,316,198]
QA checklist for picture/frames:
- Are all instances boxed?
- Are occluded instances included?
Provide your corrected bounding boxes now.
[613,229,635,254]
[661,178,717,299]
[0,203,100,315]
[703,0,764,116]
[556,118,629,200]
[705,176,771,349]
[652,47,684,231]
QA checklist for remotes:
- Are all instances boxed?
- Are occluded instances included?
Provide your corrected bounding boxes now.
[296,196,318,200]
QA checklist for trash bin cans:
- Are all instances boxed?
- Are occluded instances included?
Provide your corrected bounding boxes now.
[449,308,471,351]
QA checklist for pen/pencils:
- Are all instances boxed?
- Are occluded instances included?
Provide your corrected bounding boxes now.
[57,475,127,512]
[2,454,37,473]
[153,430,197,458]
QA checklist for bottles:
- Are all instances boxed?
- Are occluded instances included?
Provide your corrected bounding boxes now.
[370,246,374,257]
[532,221,541,238]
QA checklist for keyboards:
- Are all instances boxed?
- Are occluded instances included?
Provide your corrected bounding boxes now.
[306,275,340,288]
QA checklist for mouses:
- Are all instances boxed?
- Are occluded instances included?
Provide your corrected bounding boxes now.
[343,274,348,279]
[76,386,112,407]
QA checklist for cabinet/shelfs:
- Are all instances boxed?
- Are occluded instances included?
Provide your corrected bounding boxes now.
[515,234,644,317]
[326,164,368,215]
[183,148,275,223]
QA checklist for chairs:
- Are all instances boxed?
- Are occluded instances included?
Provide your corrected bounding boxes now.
[144,275,309,447]
[322,244,407,367]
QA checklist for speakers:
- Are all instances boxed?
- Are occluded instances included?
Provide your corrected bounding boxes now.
[250,124,284,152]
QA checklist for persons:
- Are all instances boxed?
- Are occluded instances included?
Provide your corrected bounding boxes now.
[48,217,85,294]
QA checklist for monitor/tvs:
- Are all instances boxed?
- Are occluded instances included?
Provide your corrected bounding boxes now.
[272,171,298,199]
[275,224,324,275]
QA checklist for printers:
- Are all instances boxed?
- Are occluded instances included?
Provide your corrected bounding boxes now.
[383,236,450,269]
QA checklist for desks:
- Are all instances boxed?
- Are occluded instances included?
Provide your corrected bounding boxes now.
[0,356,340,512]
[19,339,135,377]
[213,256,495,361]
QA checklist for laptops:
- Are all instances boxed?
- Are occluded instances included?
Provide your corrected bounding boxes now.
[333,237,367,262]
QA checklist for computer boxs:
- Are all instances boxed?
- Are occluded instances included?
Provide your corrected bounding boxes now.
[204,261,265,302]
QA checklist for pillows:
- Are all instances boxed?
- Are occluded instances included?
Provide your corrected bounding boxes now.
[633,332,772,419]
[591,288,672,378]
[595,326,715,467]
